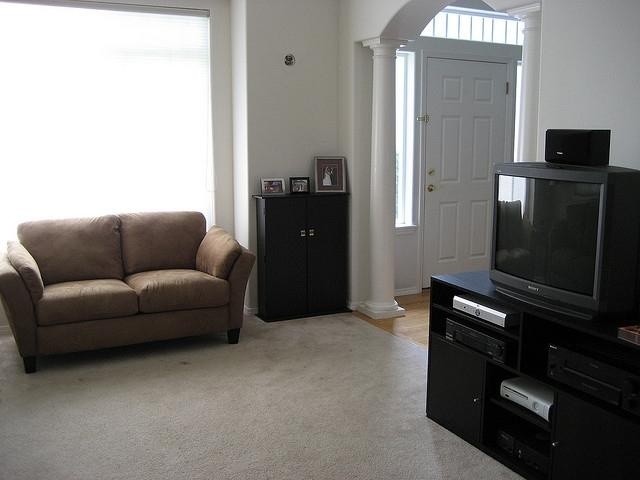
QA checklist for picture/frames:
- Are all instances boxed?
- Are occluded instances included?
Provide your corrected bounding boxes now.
[260,156,346,194]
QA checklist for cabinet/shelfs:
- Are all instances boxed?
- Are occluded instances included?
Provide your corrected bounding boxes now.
[252,192,354,324]
[425,273,640,479]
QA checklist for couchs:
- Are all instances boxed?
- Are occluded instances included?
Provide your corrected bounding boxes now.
[1,211,256,374]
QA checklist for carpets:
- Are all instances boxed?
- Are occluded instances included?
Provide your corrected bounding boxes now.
[0,310,526,480]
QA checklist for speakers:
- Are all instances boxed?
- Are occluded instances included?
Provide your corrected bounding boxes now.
[544,129,612,169]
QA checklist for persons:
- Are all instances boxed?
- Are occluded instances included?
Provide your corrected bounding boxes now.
[322,167,333,186]
[329,168,337,184]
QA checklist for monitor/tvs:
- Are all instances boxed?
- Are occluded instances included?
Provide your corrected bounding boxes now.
[487,160,639,323]
